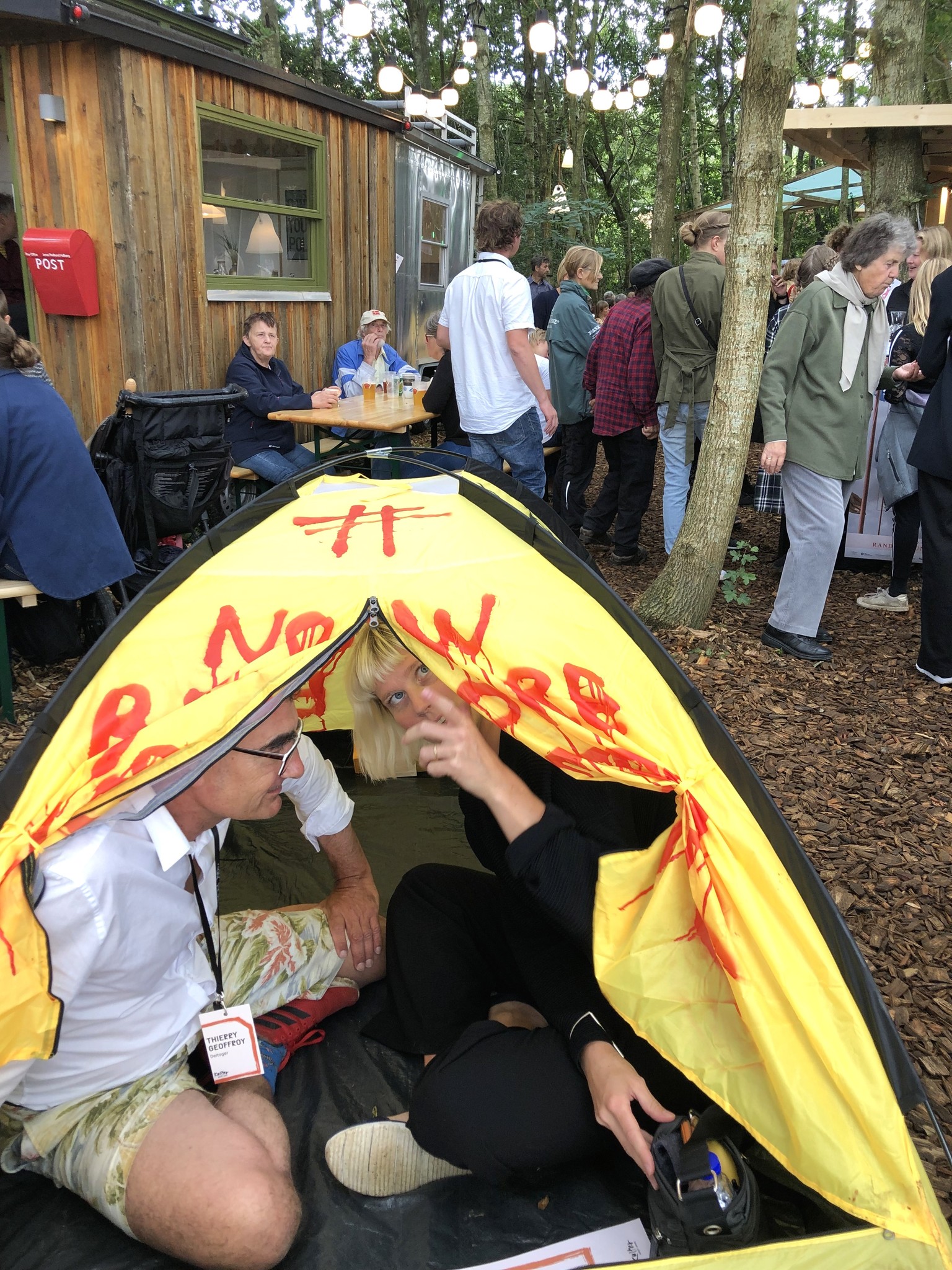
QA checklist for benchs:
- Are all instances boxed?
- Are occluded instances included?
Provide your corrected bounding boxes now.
[230,436,366,509]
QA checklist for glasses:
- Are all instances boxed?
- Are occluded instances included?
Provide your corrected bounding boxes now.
[425,334,434,343]
[230,716,304,776]
[582,266,601,273]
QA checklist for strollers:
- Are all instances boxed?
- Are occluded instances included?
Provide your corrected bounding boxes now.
[72,377,251,655]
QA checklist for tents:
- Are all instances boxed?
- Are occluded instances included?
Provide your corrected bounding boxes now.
[0,443,951,1270]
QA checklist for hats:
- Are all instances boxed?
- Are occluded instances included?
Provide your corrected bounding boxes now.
[604,291,615,301]
[628,292,636,298]
[360,309,390,325]
[625,258,674,292]
[615,294,627,303]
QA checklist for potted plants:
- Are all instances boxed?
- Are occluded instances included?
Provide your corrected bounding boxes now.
[216,228,239,275]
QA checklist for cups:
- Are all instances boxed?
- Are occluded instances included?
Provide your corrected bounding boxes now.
[385,374,401,398]
[402,373,415,398]
[324,389,339,408]
[362,377,378,405]
[384,371,396,396]
[412,381,428,411]
[393,376,403,396]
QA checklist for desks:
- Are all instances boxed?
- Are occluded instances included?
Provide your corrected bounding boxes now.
[266,391,443,479]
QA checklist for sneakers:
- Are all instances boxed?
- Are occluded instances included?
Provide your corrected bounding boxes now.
[916,656,952,684]
[254,976,361,1074]
[857,586,909,612]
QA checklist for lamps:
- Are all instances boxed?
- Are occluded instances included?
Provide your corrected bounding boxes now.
[561,121,573,168]
[547,145,570,214]
[201,203,226,219]
[38,93,65,123]
[245,137,284,254]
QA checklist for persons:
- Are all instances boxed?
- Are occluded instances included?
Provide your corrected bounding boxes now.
[0,294,137,664]
[331,310,421,479]
[436,198,566,555]
[2,691,384,1270]
[402,310,472,478]
[529,210,951,687]
[323,606,715,1196]
[225,311,342,483]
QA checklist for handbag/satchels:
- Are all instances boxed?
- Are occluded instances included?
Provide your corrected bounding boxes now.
[753,466,785,515]
[132,543,186,597]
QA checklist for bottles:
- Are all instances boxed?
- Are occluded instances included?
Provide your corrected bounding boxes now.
[686,1151,737,1213]
[887,311,907,356]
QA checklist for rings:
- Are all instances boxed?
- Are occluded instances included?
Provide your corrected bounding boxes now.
[434,744,439,760]
[767,458,772,460]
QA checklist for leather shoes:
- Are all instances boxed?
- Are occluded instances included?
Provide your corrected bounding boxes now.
[760,621,834,660]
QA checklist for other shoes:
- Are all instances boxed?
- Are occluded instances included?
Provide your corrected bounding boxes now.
[608,546,649,566]
[739,484,756,504]
[325,1115,475,1199]
[579,527,613,547]
[727,536,742,549]
[732,516,742,528]
[718,570,727,580]
